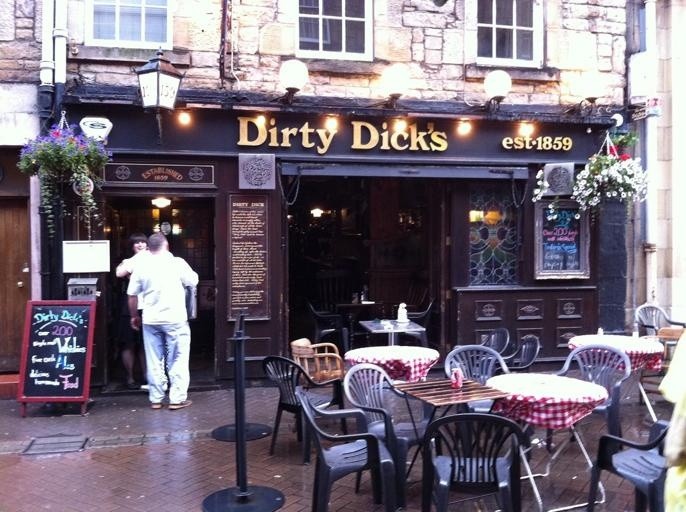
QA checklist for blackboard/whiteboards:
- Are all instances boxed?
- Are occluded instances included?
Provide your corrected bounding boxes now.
[20,300,95,403]
[534,199,591,281]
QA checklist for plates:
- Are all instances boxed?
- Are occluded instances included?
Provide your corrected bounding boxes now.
[396,320,410,322]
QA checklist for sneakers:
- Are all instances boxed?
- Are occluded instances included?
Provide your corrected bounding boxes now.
[141,383,168,391]
[152,402,163,409]
[169,400,192,409]
[127,382,139,389]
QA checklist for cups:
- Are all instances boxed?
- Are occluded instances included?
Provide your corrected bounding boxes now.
[597,328,604,336]
[397,309,408,320]
[79,116,112,145]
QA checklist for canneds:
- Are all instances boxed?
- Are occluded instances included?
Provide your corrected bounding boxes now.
[450,368,462,388]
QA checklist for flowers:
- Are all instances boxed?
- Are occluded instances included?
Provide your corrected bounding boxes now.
[568,129,651,209]
[14,110,113,244]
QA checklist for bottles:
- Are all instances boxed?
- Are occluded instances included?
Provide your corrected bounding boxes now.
[631,322,639,339]
[450,368,463,388]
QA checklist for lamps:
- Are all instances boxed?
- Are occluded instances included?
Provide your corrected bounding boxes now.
[381,64,412,107]
[279,57,309,104]
[483,68,513,114]
[129,45,188,144]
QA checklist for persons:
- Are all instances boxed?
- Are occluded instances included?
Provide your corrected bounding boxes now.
[108,232,200,410]
[656,329,686,512]
